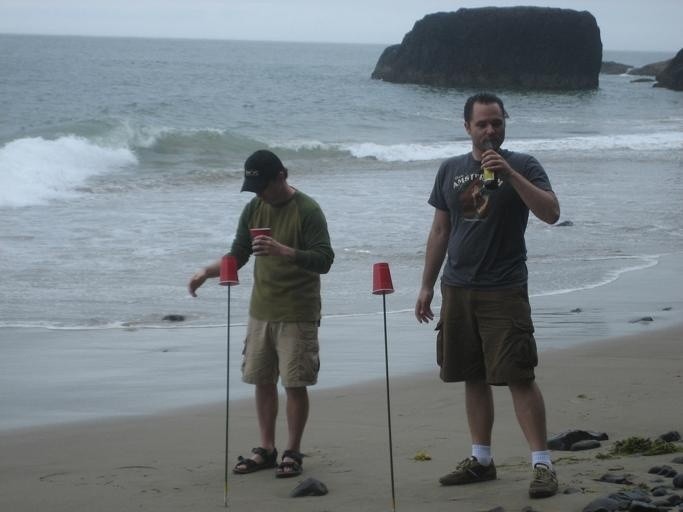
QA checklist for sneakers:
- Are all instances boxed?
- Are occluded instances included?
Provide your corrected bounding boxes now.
[529,463,558,498]
[439,456,497,485]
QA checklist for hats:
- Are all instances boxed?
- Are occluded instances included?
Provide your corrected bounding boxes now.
[241,150,285,192]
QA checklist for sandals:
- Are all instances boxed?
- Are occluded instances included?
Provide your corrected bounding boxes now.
[274,449,303,478]
[230,447,277,474]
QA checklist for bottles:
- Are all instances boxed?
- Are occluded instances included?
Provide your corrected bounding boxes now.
[483,139,498,191]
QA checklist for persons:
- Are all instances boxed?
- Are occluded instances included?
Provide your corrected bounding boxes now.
[415,95,560,501]
[187,148,339,478]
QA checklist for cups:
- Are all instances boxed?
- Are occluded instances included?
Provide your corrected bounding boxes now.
[249,228,272,240]
[372,263,395,295]
[218,255,240,286]
